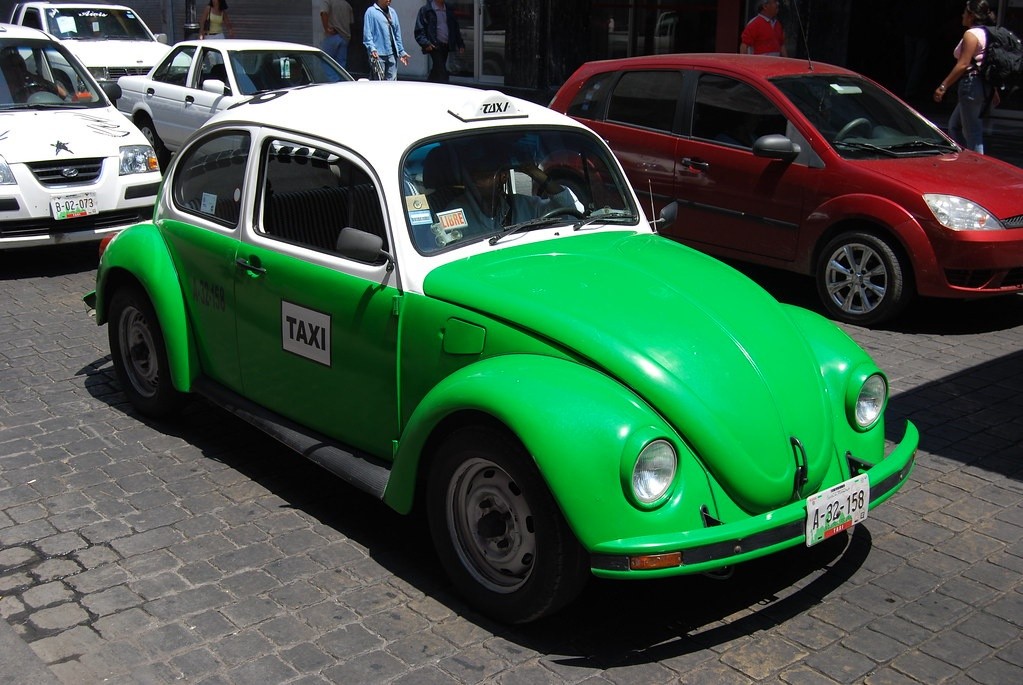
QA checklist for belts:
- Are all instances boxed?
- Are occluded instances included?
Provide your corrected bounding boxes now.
[205,33,217,35]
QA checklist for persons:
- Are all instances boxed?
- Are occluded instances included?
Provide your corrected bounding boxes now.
[363,0,411,81]
[932,0,1002,154]
[198,0,233,39]
[414,0,465,82]
[320,0,354,82]
[740,0,787,57]
[442,146,585,243]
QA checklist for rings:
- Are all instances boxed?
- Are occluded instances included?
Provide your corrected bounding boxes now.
[936,95,938,98]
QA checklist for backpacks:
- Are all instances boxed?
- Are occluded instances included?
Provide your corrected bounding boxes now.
[970,25,1023,88]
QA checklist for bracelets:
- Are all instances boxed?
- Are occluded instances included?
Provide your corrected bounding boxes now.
[542,173,551,188]
[939,84,947,92]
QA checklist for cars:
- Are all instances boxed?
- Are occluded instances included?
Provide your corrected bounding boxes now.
[0,25,161,252]
[81,74,921,626]
[116,38,372,178]
[539,52,1023,323]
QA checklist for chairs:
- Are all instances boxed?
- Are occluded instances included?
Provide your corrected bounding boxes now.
[210,64,228,83]
[422,146,500,242]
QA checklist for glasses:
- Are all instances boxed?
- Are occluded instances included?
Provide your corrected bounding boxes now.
[7,62,25,70]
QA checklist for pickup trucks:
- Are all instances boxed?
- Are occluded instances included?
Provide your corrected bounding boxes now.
[0,1,199,104]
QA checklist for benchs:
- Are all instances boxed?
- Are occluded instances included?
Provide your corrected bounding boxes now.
[186,182,421,251]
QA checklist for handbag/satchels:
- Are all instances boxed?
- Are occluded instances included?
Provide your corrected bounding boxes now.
[204,5,211,31]
